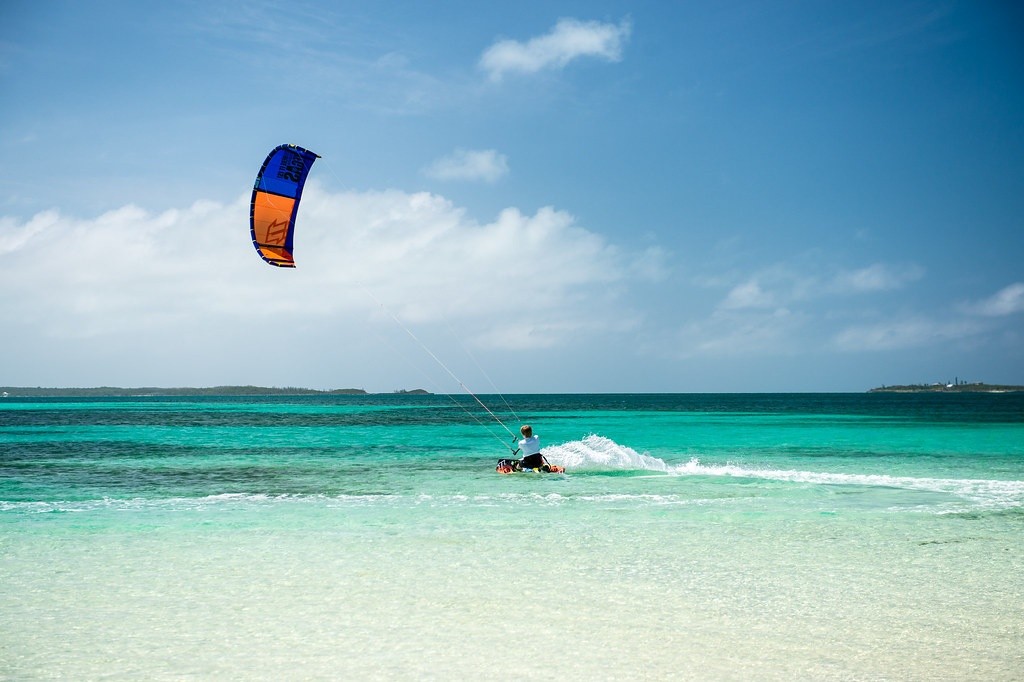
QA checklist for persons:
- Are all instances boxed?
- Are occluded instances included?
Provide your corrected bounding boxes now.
[516,425,543,468]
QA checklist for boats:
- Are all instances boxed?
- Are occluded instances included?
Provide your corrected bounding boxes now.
[495,459,566,474]
